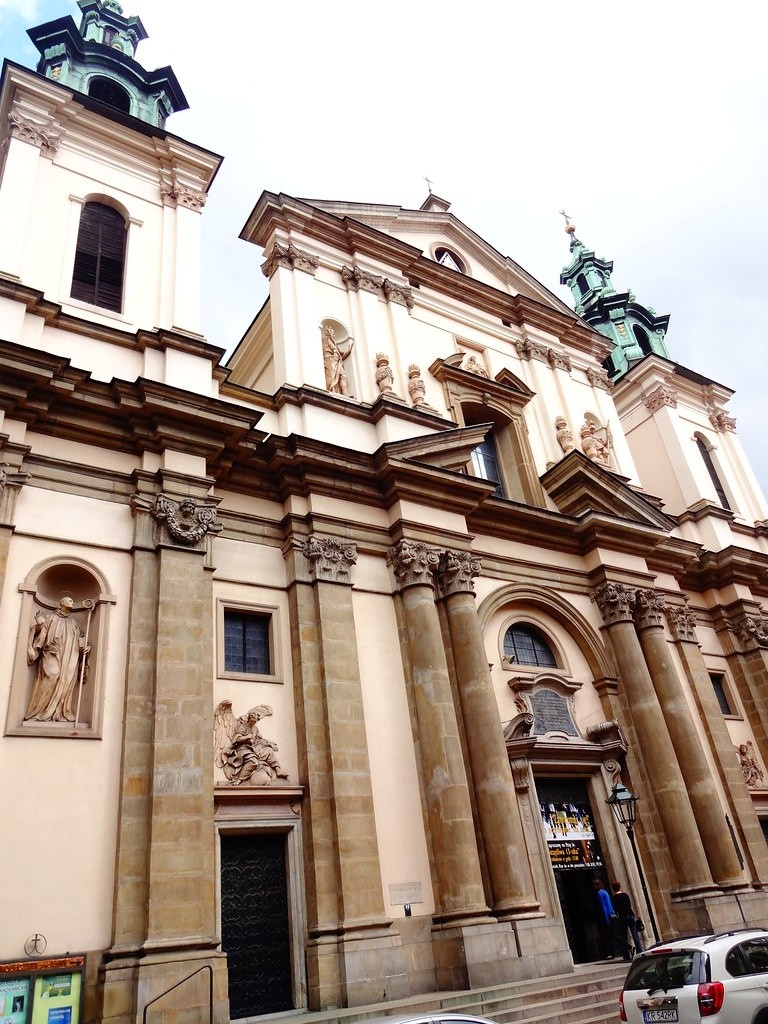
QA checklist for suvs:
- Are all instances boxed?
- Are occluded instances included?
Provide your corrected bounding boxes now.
[616,926,768,1024]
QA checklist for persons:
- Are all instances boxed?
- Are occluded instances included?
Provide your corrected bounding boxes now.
[322,325,354,400]
[466,356,489,379]
[593,879,636,960]
[231,712,289,785]
[589,421,609,463]
[24,596,91,723]
[740,743,761,785]
[609,880,643,960]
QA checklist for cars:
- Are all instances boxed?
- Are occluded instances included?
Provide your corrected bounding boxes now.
[350,1011,502,1024]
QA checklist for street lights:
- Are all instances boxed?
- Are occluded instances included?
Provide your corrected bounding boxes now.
[604,780,662,944]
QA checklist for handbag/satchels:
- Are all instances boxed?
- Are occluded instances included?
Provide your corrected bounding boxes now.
[635,918,645,932]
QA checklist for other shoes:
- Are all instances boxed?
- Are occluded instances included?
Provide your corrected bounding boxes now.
[606,955,614,960]
[622,954,630,960]
